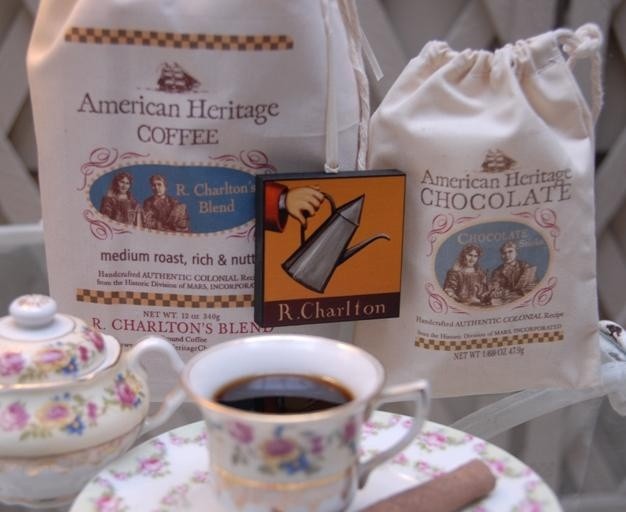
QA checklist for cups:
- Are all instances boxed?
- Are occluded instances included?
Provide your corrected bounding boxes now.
[180,334,430,511]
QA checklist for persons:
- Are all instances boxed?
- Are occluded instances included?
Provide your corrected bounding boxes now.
[101,173,189,233]
[444,242,540,311]
[265,181,325,231]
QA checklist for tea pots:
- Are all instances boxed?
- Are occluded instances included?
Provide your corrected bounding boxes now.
[0,294,188,511]
[282,191,392,293]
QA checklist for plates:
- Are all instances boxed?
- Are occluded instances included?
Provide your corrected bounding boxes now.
[68,409,562,512]
